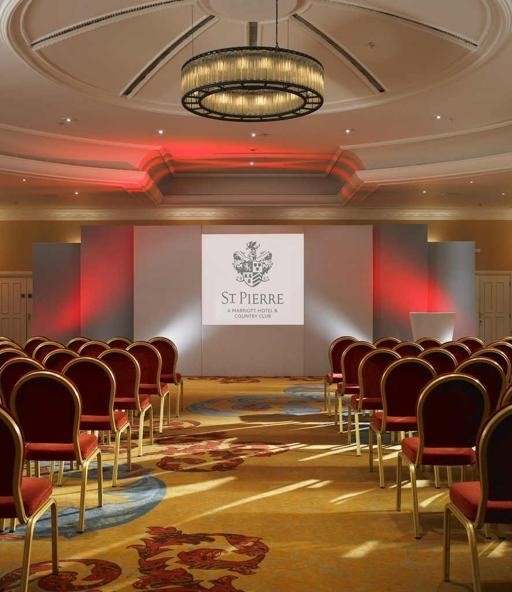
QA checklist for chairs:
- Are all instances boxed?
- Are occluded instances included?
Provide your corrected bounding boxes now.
[0,337,12,342]
[416,336,441,350]
[456,358,506,417]
[324,335,358,417]
[0,408,59,592]
[370,358,437,489]
[148,337,184,417]
[443,406,512,592]
[471,349,510,384]
[486,341,511,361]
[32,342,66,364]
[42,349,80,373]
[440,341,471,366]
[107,337,132,350]
[66,337,90,352]
[500,337,512,344]
[458,335,485,355]
[373,337,401,350]
[10,370,104,533]
[502,385,512,408]
[335,342,377,434]
[78,341,110,359]
[126,342,172,435]
[418,348,459,376]
[1,358,55,488]
[61,357,131,488]
[392,342,425,358]
[0,348,28,368]
[0,341,22,351]
[346,349,401,457]
[397,375,490,538]
[23,336,50,357]
[97,349,153,457]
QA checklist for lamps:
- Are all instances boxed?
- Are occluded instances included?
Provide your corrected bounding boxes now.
[179,20,324,123]
